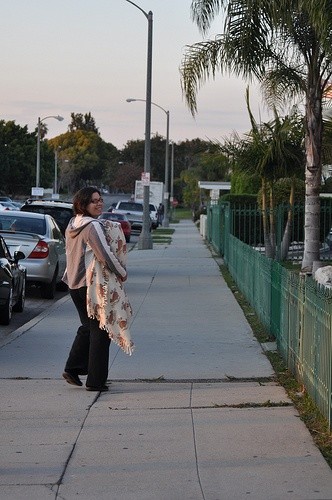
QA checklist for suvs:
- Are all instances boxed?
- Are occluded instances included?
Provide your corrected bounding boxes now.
[8,199,77,238]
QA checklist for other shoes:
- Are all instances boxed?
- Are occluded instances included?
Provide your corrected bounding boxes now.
[86,384,109,391]
[62,371,82,386]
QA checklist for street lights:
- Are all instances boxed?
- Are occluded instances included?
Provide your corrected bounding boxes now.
[119,161,134,200]
[143,132,174,221]
[126,98,171,228]
[55,145,61,194]
[35,114,65,199]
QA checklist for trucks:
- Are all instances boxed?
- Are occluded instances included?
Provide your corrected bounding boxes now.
[130,179,164,210]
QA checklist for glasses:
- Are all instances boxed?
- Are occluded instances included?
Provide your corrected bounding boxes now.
[90,198,104,204]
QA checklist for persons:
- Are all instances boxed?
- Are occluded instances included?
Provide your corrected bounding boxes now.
[157,203,164,225]
[200,193,206,208]
[61,186,127,391]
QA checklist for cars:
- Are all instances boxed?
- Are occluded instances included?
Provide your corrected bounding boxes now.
[0,234,27,326]
[0,209,67,299]
[0,195,54,211]
[149,203,161,228]
[110,200,153,232]
[98,212,134,243]
